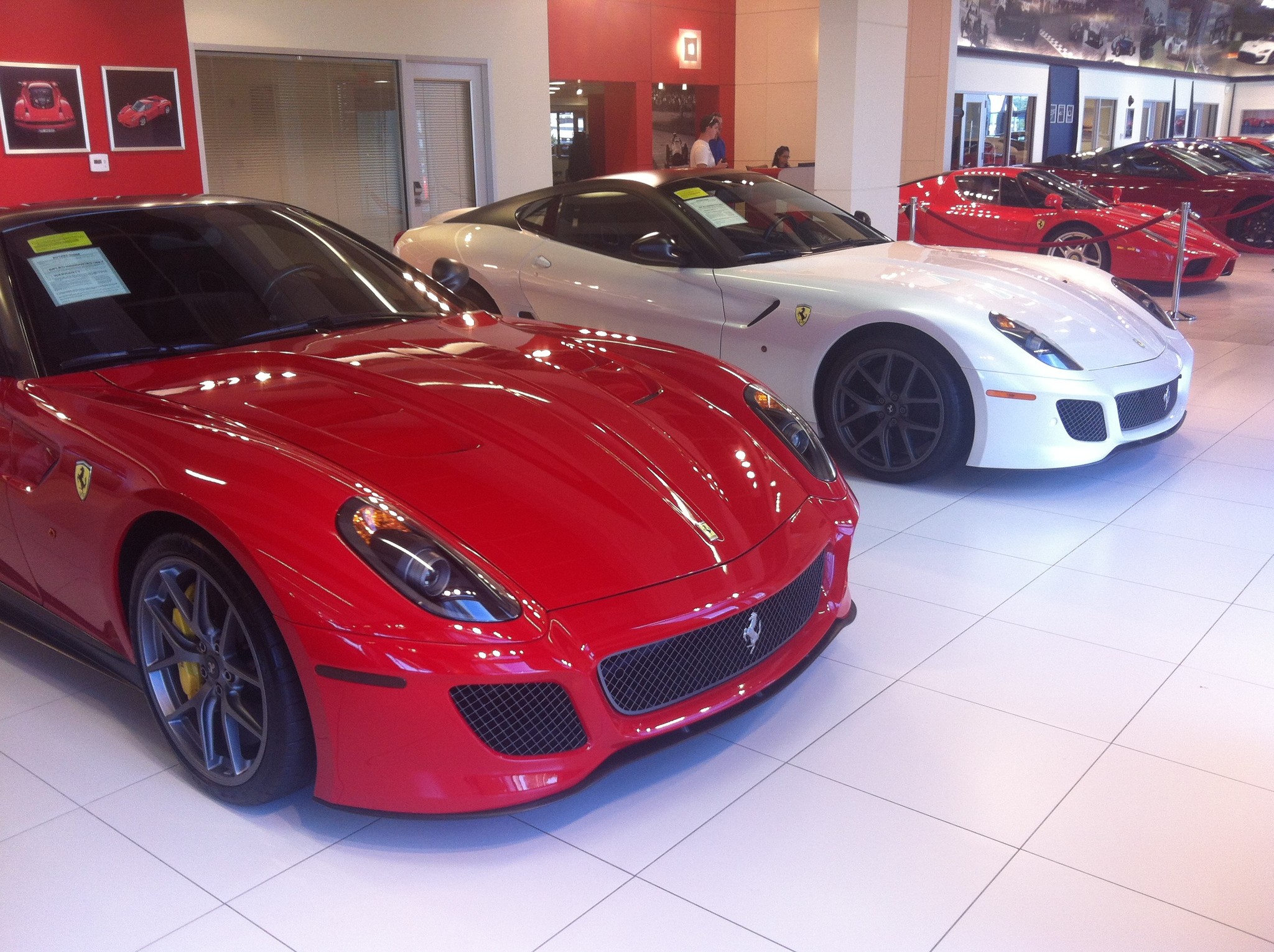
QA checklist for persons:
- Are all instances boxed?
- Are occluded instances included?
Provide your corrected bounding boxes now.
[771,146,794,170]
[708,112,726,167]
[689,115,727,169]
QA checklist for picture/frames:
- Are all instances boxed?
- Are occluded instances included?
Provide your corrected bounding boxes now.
[1237,108,1274,137]
[1124,108,1135,139]
[1172,109,1186,136]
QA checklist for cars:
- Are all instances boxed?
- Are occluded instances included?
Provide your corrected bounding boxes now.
[959,5,988,46]
[551,142,574,184]
[1069,6,1189,60]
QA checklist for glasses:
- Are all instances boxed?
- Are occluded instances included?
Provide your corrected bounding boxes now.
[780,155,790,159]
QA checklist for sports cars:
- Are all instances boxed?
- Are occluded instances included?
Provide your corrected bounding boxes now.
[895,168,1240,293]
[950,129,1026,171]
[386,167,1198,485]
[1207,136,1274,162]
[1237,33,1274,65]
[2,195,862,818]
[116,95,172,129]
[1242,117,1274,128]
[13,80,77,136]
[1039,138,1274,257]
